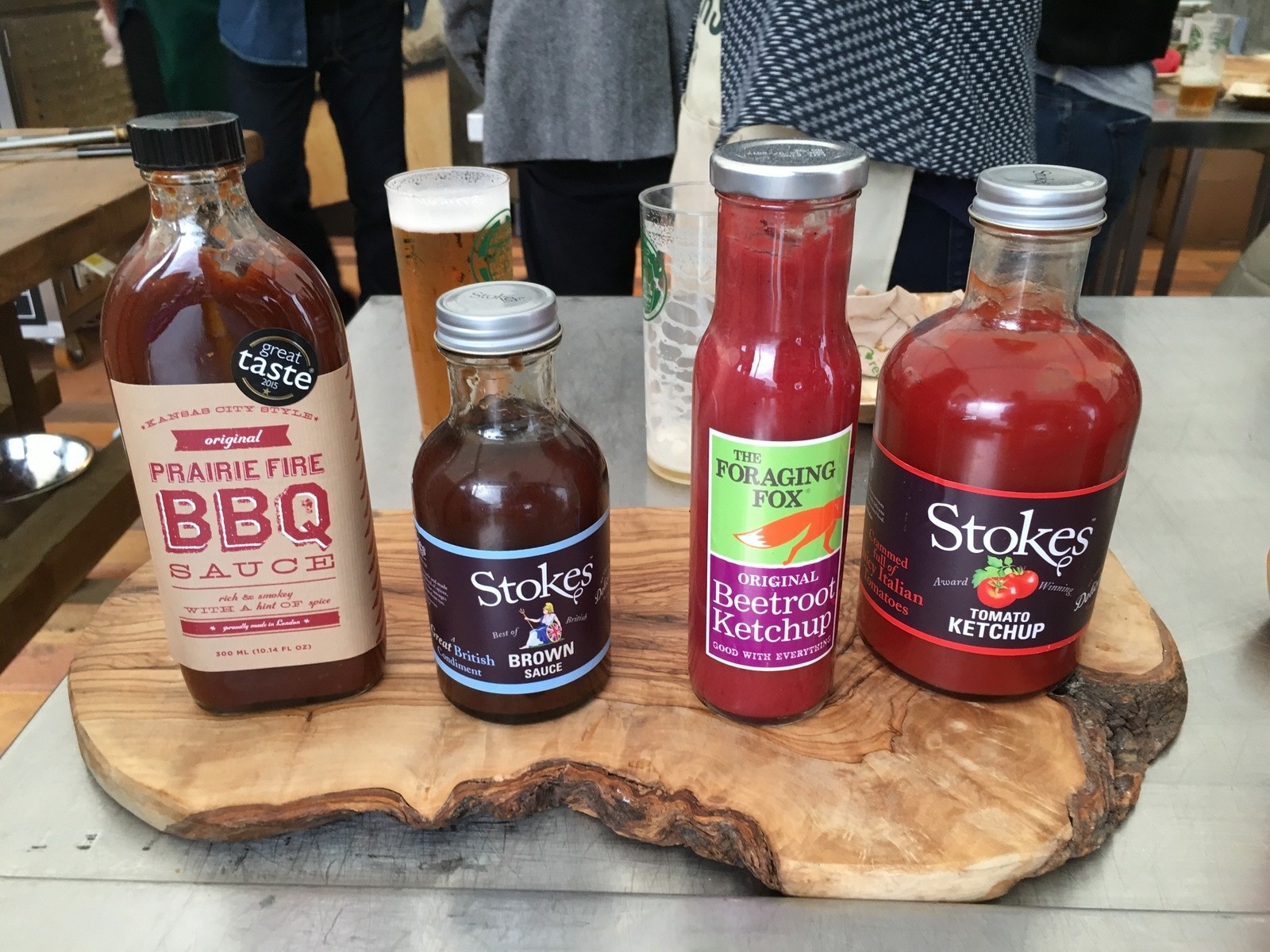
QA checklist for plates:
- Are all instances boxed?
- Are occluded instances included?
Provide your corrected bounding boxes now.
[1155,70,1177,82]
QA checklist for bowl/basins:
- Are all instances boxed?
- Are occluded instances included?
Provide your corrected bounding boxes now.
[0,432,97,538]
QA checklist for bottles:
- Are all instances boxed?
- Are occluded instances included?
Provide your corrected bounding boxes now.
[686,137,870,718]
[856,163,1144,697]
[413,280,612,719]
[99,110,385,714]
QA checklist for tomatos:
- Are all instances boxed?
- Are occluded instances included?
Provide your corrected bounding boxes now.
[977,565,1038,609]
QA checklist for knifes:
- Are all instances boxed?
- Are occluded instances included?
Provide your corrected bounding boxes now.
[1,144,131,161]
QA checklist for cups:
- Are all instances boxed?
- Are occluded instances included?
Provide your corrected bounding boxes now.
[1179,11,1234,111]
[639,181,718,486]
[385,168,516,445]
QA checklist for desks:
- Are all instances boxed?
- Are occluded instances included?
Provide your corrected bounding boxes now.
[0,128,262,679]
[1096,82,1270,296]
[1,294,1269,951]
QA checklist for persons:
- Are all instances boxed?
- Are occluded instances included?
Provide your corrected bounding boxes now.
[99,1,1183,320]
